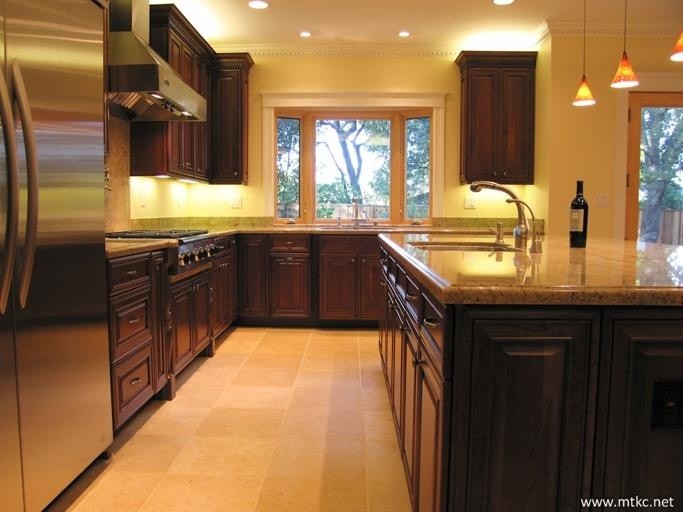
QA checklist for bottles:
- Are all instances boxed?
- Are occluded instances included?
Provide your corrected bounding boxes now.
[569,179,588,248]
[569,246,586,285]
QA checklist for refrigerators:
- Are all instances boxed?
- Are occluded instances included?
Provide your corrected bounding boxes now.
[0,1,115,512]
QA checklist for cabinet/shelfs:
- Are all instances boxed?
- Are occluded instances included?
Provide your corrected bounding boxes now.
[214,53,254,184]
[194,37,215,184]
[454,51,538,184]
[106,248,172,440]
[172,239,215,377]
[267,235,313,326]
[237,233,269,325]
[317,236,379,326]
[214,236,239,340]
[591,305,682,511]
[130,3,194,180]
[442,304,591,511]
[377,247,417,500]
[413,292,449,512]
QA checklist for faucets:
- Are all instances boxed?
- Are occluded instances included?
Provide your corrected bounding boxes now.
[469,181,529,249]
[505,198,542,253]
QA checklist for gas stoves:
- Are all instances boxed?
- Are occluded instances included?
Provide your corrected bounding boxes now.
[105,228,208,240]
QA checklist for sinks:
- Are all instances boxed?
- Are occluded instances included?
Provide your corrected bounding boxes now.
[409,242,514,252]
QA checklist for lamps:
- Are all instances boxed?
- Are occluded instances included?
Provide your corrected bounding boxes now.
[670,31,683,62]
[610,1,640,87]
[572,1,598,106]
